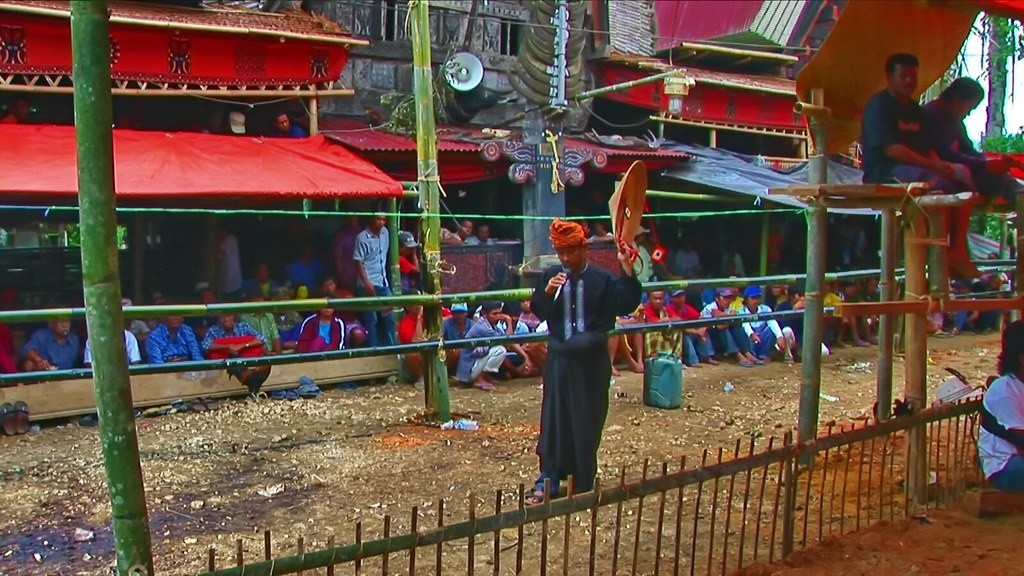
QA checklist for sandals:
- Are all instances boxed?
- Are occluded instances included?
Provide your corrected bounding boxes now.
[524,481,560,506]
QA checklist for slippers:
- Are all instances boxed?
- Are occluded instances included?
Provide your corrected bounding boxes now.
[272,389,299,400]
[0,401,30,436]
[296,376,321,396]
[188,397,219,413]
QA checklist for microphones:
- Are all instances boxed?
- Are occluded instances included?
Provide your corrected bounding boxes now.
[554,268,572,302]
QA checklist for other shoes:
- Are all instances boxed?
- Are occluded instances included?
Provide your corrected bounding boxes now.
[736,357,764,367]
[473,382,497,391]
[686,363,702,368]
[784,359,794,364]
[30,424,40,433]
[336,382,356,392]
[699,357,719,365]
[852,340,870,347]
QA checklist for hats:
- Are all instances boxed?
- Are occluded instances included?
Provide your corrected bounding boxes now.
[741,286,762,297]
[609,160,647,250]
[350,326,366,348]
[481,302,501,311]
[450,302,468,313]
[936,376,983,405]
[548,218,588,249]
[270,286,292,301]
[296,285,308,298]
[668,290,685,297]
[398,232,418,248]
[715,287,733,297]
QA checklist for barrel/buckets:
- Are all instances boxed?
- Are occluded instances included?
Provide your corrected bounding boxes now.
[643,351,682,408]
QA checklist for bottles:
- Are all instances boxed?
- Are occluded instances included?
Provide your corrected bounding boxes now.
[848,139,863,169]
[724,382,734,393]
[901,471,936,492]
[75,531,95,541]
[453,418,479,430]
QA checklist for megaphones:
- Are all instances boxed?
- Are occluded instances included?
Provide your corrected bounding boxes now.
[445,51,484,91]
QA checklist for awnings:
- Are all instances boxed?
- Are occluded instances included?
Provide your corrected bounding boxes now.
[318,116,696,160]
[655,140,909,217]
[0,123,404,199]
[965,228,1014,260]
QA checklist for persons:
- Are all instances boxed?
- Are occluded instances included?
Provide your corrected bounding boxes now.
[0,94,307,139]
[976,321,1024,492]
[524,217,642,505]
[609,223,902,376]
[927,252,1010,335]
[0,209,548,390]
[861,52,1024,277]
